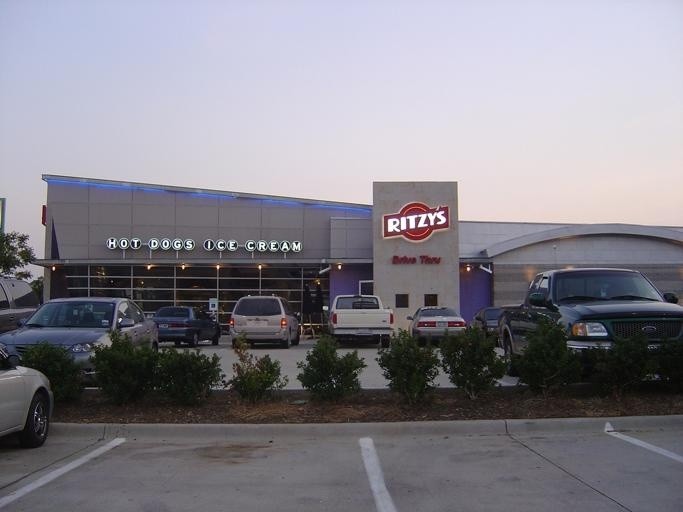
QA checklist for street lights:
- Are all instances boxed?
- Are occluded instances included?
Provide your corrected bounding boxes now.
[552,246,558,270]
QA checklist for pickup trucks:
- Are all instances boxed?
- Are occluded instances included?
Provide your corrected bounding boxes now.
[497,267,683,381]
[321,295,393,348]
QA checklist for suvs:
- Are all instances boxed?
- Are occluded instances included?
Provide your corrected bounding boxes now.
[229,293,299,349]
[0,277,39,332]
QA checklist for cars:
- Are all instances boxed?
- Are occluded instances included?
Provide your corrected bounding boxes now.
[471,306,502,340]
[405,306,465,340]
[0,297,159,388]
[0,347,55,448]
[149,306,221,345]
